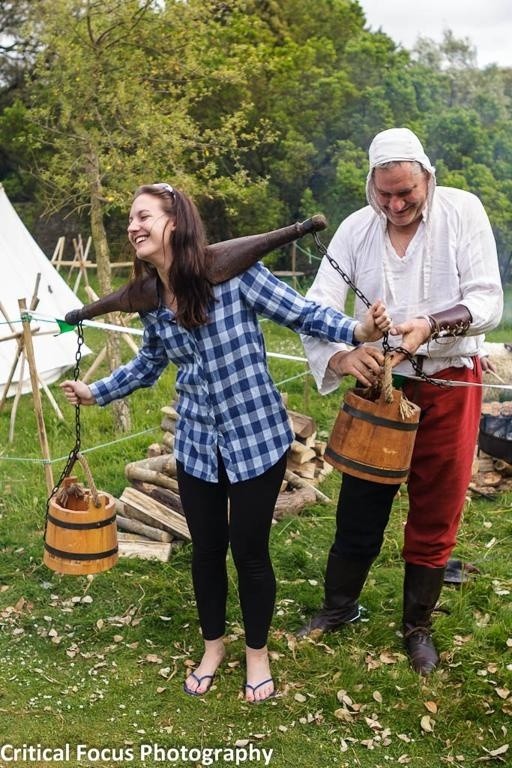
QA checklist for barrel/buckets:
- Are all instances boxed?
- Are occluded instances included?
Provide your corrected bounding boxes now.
[44,452,119,575]
[324,351,422,484]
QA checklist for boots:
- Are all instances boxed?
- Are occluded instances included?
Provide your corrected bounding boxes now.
[293,543,379,643]
[402,563,447,676]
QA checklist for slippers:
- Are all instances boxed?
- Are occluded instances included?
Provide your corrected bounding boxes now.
[183,660,216,695]
[242,662,276,704]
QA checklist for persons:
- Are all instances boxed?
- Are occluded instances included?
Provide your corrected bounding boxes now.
[58,183,392,703]
[296,127,505,677]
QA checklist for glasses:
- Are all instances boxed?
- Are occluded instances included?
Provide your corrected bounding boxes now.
[152,182,176,205]
